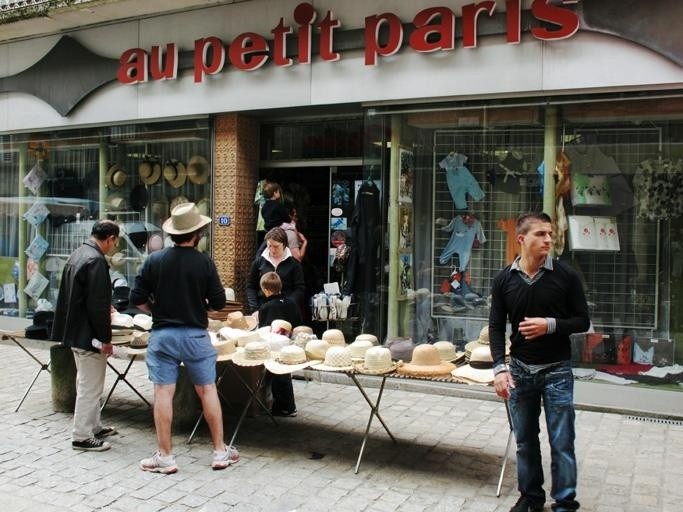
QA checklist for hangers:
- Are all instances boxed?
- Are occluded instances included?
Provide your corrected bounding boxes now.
[446,145,454,156]
[448,265,457,276]
[466,209,475,218]
[365,166,374,185]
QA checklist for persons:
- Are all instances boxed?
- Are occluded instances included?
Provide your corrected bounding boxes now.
[278,204,308,261]
[489,212,590,512]
[258,272,304,417]
[245,227,305,325]
[256,184,291,258]
[128,203,240,475]
[49,219,119,453]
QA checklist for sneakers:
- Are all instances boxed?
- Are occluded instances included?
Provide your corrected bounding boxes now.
[70,437,111,451]
[139,450,178,474]
[270,405,299,418]
[509,494,545,512]
[94,425,117,440]
[209,444,243,469]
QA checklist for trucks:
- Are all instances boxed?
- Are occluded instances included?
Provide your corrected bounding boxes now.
[0,194,163,310]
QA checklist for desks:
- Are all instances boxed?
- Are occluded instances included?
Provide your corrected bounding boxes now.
[1,336,521,498]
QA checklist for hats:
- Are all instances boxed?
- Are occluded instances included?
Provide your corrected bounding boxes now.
[105,156,212,315]
[206,311,512,386]
[222,287,242,305]
[24,311,153,355]
